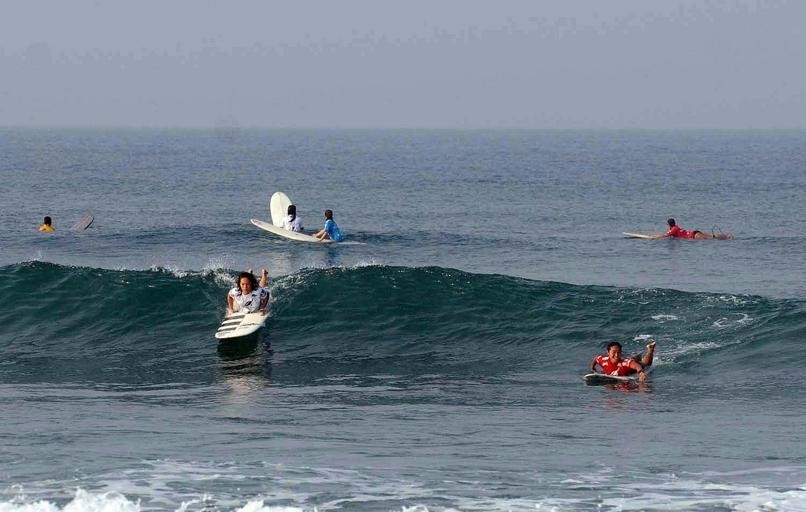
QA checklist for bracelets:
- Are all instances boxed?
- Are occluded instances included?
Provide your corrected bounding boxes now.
[637,370,644,374]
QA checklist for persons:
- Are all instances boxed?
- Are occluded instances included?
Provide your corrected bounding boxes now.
[312,210,342,242]
[649,218,736,240]
[604,381,654,395]
[226,268,270,315]
[280,205,303,232]
[591,341,656,381]
[39,216,55,232]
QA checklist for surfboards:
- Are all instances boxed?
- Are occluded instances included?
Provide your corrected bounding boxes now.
[585,373,638,382]
[270,192,293,228]
[622,232,670,239]
[214,312,267,338]
[71,216,94,230]
[250,219,335,242]
